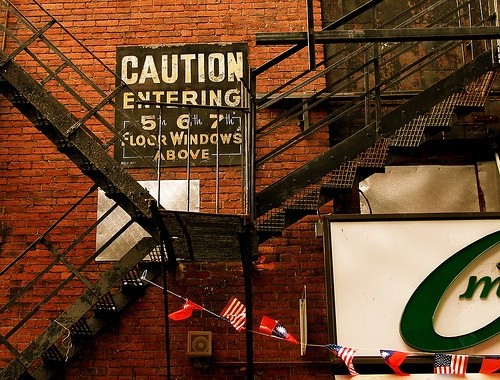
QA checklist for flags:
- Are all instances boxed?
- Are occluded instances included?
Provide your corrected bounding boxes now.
[480,357,500,374]
[219,294,247,333]
[166,299,205,320]
[260,316,299,344]
[433,353,468,377]
[327,344,360,376]
[379,349,410,377]
